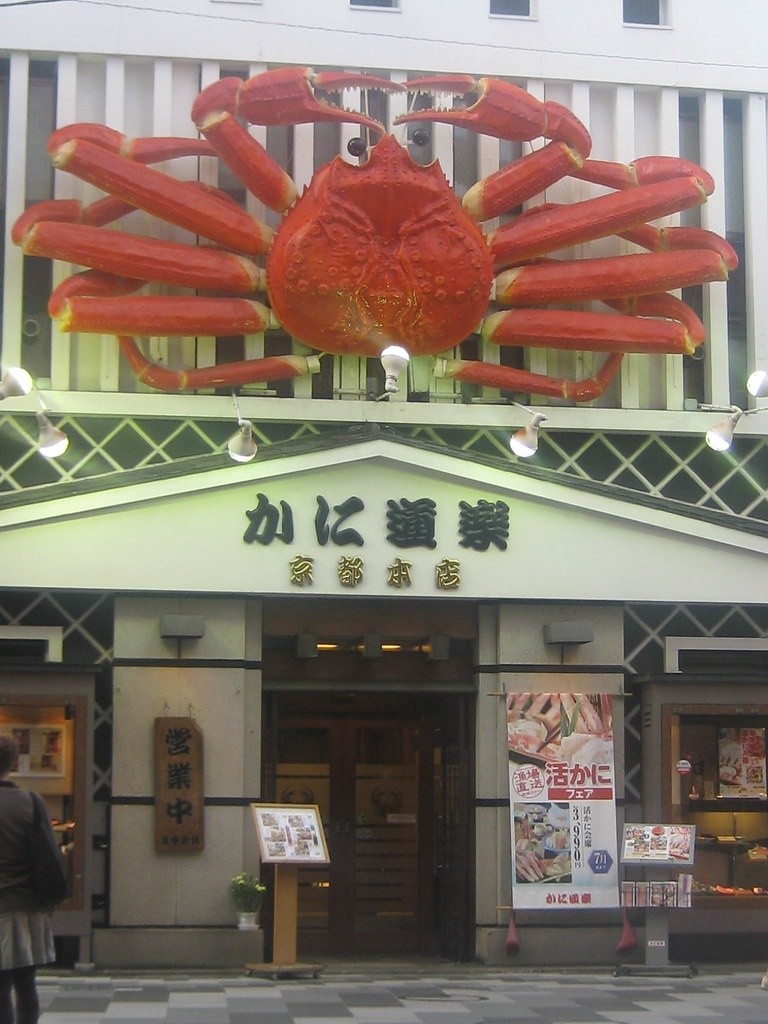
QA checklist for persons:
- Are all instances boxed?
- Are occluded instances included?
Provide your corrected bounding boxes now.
[0,732,70,1024]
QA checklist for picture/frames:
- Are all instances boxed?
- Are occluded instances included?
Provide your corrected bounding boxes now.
[0,723,68,779]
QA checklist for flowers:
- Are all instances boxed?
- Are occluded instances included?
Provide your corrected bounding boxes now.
[230,872,267,914]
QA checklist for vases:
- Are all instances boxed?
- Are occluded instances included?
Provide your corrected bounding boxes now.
[238,913,261,932]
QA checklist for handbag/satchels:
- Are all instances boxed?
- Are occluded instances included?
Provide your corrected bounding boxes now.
[28,790,75,913]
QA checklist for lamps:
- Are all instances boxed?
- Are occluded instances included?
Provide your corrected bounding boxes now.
[374,344,410,401]
[696,402,743,453]
[158,613,207,640]
[32,387,69,457]
[542,620,594,645]
[227,387,258,463]
[361,633,386,661]
[430,633,452,661]
[505,397,548,458]
[0,367,33,400]
[296,632,320,658]
[741,371,768,414]
